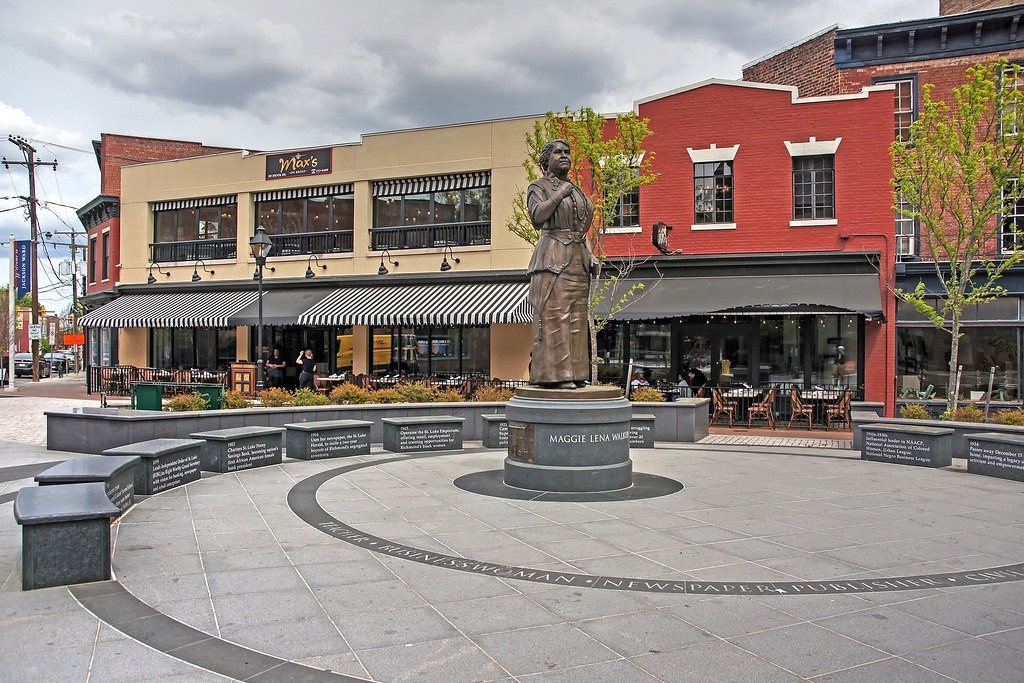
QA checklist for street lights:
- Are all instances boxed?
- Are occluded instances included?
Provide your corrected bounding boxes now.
[248,224,272,392]
[30,230,53,383]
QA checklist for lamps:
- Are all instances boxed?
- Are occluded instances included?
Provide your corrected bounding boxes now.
[192,260,215,281]
[305,254,327,278]
[148,263,170,284]
[441,246,460,271]
[378,250,399,275]
[254,263,275,280]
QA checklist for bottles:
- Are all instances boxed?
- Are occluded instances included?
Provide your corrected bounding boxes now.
[657,380,661,389]
[669,382,673,390]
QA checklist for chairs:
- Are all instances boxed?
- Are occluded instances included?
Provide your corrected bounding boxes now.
[459,376,503,399]
[748,383,815,430]
[102,366,228,397]
[313,373,331,395]
[698,383,737,427]
[826,386,852,432]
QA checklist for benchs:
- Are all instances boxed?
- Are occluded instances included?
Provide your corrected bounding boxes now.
[482,413,508,448]
[858,423,955,468]
[629,414,656,448]
[283,418,375,459]
[963,433,1024,482]
[34,456,142,523]
[190,425,286,473]
[380,415,465,453]
[102,437,207,495]
[14,481,121,591]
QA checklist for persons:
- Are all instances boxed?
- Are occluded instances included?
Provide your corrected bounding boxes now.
[631,368,650,390]
[265,349,286,387]
[296,350,317,390]
[678,368,709,398]
[834,346,848,389]
[527,139,602,392]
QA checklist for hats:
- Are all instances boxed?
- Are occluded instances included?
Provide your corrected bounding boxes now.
[635,369,646,373]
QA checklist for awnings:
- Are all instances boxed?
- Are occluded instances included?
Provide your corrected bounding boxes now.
[511,273,884,321]
[77,290,268,326]
[228,290,334,325]
[297,282,530,325]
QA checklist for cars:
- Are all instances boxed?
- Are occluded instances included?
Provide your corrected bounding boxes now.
[14,352,50,379]
[43,349,82,373]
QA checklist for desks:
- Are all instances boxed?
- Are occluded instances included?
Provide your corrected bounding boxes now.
[656,388,679,402]
[432,380,462,388]
[370,379,396,389]
[721,390,763,423]
[316,378,345,397]
[802,392,837,426]
